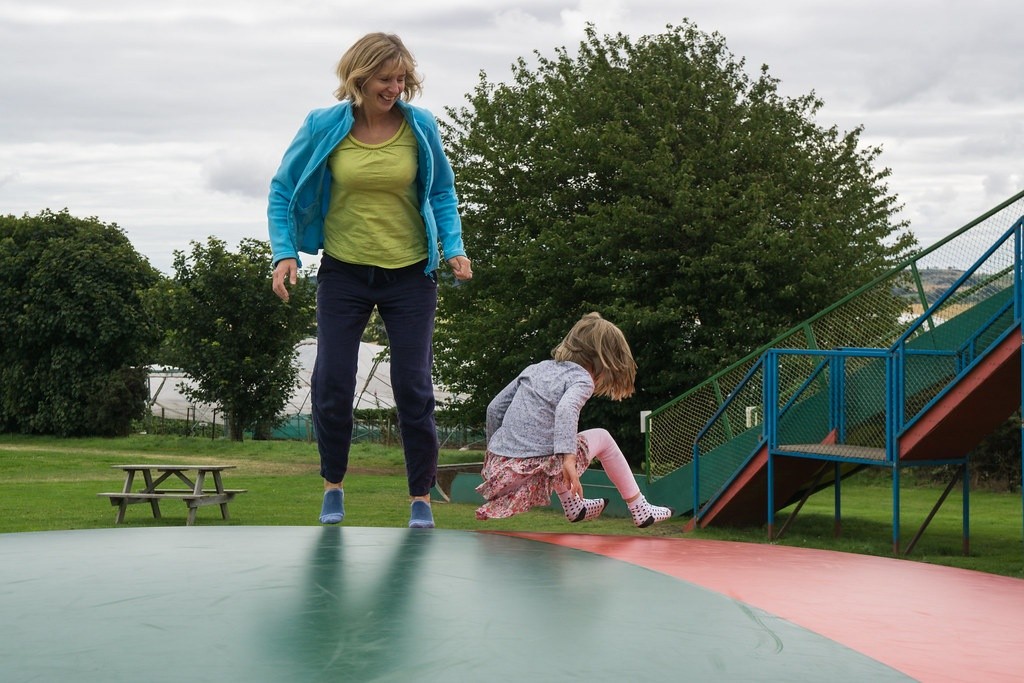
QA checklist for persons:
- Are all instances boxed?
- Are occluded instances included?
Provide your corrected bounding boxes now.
[267,32,472,528]
[475,312,675,528]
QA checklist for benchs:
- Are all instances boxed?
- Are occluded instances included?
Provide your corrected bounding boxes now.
[96,488,248,508]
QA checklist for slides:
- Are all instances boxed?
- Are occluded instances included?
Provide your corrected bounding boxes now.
[427,178,1024,510]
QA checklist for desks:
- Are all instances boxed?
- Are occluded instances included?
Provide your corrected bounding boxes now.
[108,464,237,526]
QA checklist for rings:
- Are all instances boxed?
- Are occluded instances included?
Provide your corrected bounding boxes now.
[470,271,473,273]
